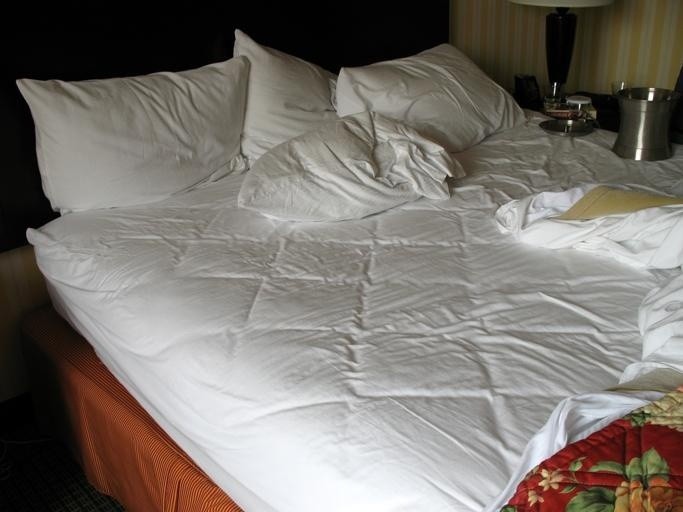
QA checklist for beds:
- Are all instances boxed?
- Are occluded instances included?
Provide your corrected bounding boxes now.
[19,105,682,512]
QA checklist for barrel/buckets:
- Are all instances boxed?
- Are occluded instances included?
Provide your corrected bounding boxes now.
[613,87,680,160]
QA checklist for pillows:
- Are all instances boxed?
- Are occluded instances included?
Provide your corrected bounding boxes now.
[16,56,249,214]
[328,43,527,157]
[236,107,466,219]
[232,29,337,171]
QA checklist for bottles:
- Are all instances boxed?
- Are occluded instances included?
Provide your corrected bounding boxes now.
[567,97,591,133]
